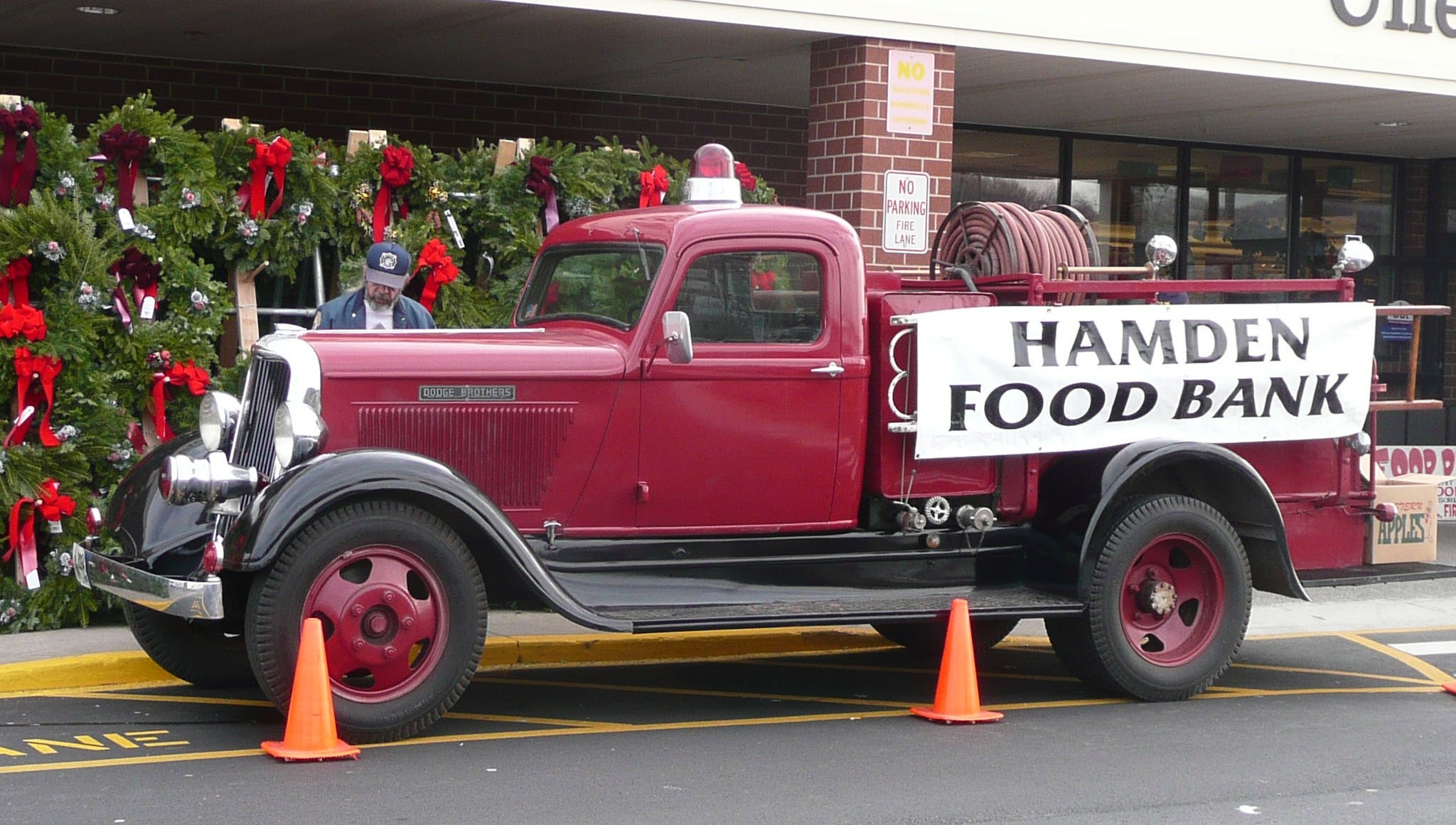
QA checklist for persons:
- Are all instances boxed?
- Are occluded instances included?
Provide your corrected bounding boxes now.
[312,242,436,330]
[1155,266,1190,304]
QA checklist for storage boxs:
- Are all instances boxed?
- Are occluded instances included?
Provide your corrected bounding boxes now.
[1360,453,1456,565]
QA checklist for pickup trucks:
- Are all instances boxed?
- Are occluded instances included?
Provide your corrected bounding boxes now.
[72,141,1392,748]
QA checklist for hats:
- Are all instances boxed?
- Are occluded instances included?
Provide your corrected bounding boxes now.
[366,242,409,288]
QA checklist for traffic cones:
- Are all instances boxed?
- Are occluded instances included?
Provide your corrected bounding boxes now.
[911,598,1004,725]
[261,618,361,765]
